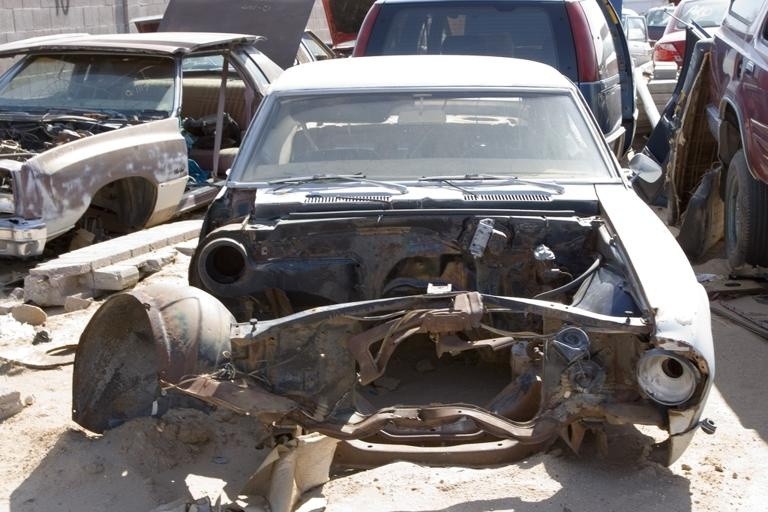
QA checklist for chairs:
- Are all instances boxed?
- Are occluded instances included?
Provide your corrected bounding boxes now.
[107,79,260,174]
